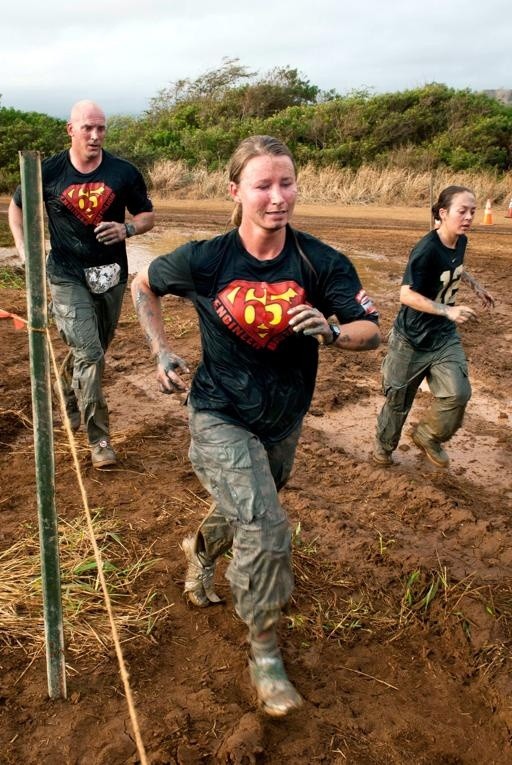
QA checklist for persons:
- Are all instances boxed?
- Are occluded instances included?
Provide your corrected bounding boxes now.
[7,101,155,470]
[132,135,382,718]
[370,184,495,468]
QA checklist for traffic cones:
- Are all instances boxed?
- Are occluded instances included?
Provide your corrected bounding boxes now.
[505,197,512,217]
[480,198,495,225]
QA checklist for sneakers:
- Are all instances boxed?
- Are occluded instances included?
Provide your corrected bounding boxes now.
[245,648,303,718]
[183,538,226,608]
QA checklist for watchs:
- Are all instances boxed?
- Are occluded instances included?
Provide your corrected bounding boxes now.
[124,222,136,236]
[328,323,341,346]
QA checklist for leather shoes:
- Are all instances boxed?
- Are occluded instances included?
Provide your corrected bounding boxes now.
[411,431,449,468]
[53,381,81,430]
[372,437,392,465]
[91,437,117,468]
